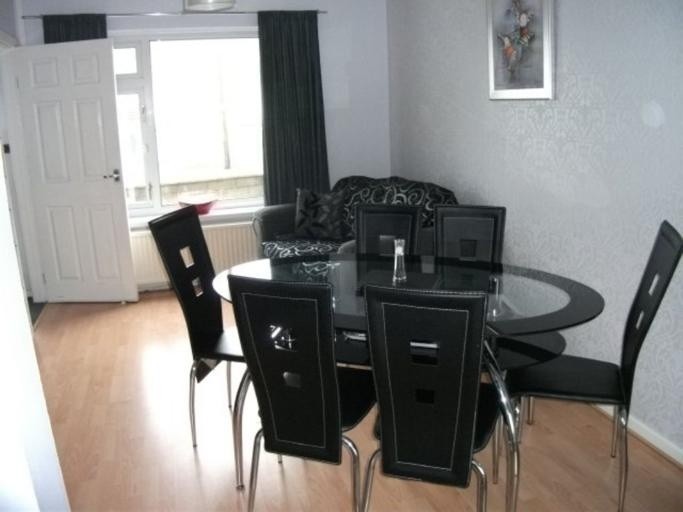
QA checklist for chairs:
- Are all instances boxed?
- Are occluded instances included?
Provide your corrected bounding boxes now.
[360,286,500,512]
[501,220,683,512]
[432,203,506,263]
[148,205,247,448]
[354,202,422,256]
[224,272,377,512]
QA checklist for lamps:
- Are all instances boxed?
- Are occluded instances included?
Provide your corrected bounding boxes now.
[183,0,235,13]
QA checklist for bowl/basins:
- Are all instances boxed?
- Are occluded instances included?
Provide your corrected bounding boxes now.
[177,192,218,215]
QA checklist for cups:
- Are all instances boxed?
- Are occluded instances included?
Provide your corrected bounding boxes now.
[393,239,408,281]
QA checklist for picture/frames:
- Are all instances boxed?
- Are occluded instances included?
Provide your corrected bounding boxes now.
[485,0,555,99]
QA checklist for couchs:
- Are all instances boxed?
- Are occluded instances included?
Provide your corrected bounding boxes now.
[250,175,458,258]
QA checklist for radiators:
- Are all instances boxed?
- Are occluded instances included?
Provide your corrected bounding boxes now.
[130,221,257,286]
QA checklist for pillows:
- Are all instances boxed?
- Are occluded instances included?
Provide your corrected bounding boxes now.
[294,187,346,241]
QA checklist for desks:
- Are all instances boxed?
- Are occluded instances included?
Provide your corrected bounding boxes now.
[212,253,605,512]
